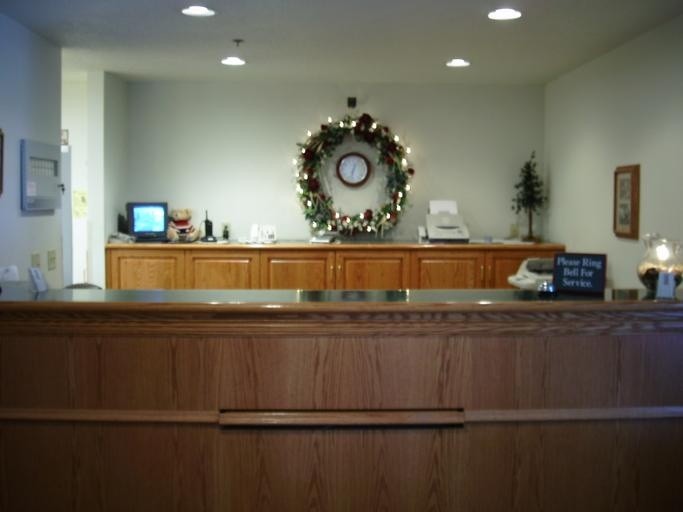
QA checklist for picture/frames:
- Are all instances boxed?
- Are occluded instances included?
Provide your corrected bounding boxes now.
[612,164,639,240]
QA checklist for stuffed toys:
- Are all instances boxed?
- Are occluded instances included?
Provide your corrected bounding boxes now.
[166,207,200,243]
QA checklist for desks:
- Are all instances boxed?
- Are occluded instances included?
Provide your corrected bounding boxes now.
[0,288,683,511]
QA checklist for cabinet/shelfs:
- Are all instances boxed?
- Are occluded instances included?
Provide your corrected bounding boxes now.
[260,250,410,289]
[410,251,565,290]
[184,250,260,289]
[106,247,185,289]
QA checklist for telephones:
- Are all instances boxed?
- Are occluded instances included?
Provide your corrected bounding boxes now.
[201,209,217,242]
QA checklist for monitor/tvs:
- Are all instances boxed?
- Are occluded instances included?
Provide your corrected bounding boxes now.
[126,202,168,241]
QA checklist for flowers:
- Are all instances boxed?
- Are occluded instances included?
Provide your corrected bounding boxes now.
[292,113,417,238]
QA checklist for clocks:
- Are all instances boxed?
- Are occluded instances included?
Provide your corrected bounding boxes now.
[337,152,370,187]
